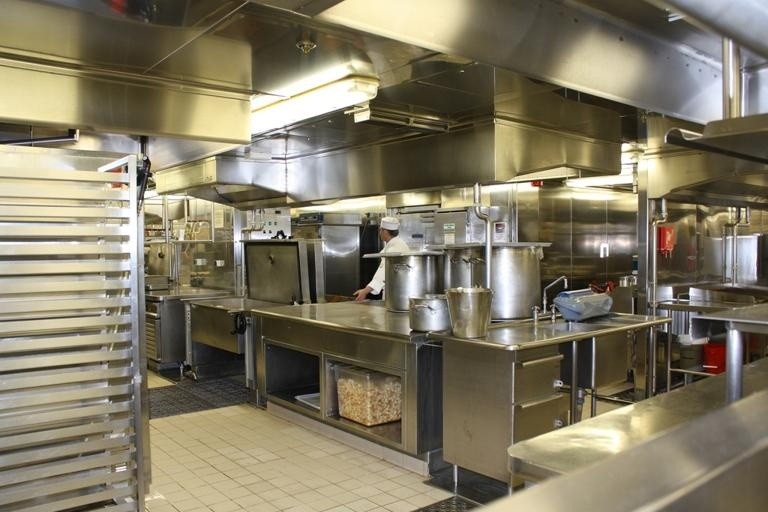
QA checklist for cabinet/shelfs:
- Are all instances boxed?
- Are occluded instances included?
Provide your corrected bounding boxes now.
[181,296,287,390]
[427,311,671,494]
[145,288,229,375]
[251,297,454,478]
[659,300,765,394]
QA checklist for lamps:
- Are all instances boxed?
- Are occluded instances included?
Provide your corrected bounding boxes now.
[250,76,380,136]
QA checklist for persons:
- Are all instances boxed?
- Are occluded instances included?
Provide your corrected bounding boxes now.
[352,216,410,301]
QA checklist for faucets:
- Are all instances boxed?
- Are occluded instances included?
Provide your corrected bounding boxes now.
[543,274,567,314]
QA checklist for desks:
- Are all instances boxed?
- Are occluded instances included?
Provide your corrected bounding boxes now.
[508,356,767,493]
[692,302,768,409]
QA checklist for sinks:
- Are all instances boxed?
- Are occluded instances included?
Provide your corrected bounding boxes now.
[533,319,616,336]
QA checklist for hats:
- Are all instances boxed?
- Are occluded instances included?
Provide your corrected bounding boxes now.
[381,216,400,230]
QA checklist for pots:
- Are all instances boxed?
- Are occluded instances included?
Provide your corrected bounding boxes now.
[384,254,444,313]
[444,246,543,320]
[409,293,450,333]
